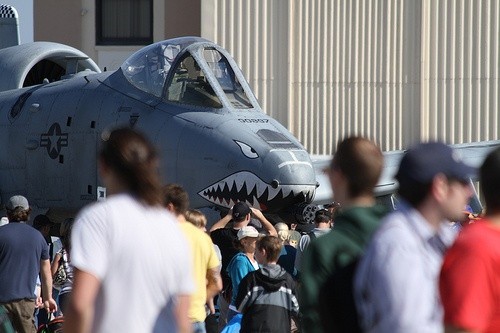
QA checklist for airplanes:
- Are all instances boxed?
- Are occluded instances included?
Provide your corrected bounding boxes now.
[0,4,500,232]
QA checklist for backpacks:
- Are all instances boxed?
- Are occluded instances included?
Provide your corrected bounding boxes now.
[205,266,232,333]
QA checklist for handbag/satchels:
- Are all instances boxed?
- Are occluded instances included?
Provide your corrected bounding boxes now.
[54,248,69,284]
[36,311,64,333]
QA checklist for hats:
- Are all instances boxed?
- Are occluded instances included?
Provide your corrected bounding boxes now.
[237,226,258,240]
[315,210,332,228]
[0,217,9,226]
[395,143,480,188]
[233,202,251,218]
[7,195,29,210]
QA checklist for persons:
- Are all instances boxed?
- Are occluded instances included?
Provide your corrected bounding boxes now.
[298,135,392,333]
[62,127,198,333]
[440,147,500,333]
[355,141,480,333]
[0,184,473,333]
